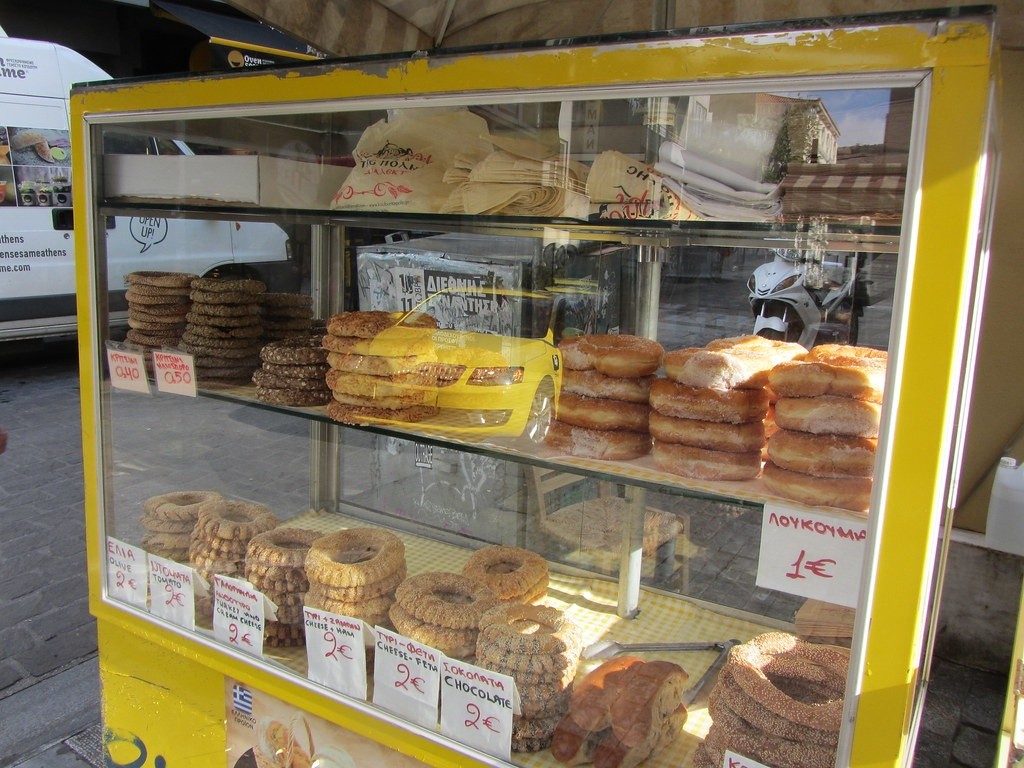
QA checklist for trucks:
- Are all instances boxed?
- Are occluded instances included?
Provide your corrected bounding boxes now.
[0,33,303,345]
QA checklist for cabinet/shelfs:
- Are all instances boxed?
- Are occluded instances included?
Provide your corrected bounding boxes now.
[71,1,1023,763]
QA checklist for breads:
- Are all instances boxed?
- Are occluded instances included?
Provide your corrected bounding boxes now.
[135,489,587,754]
[691,632,849,768]
[527,333,893,512]
[122,268,511,427]
[550,655,690,768]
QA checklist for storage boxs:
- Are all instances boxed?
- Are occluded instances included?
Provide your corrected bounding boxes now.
[101,152,356,211]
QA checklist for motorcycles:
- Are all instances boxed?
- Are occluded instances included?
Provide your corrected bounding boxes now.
[746,238,873,349]
